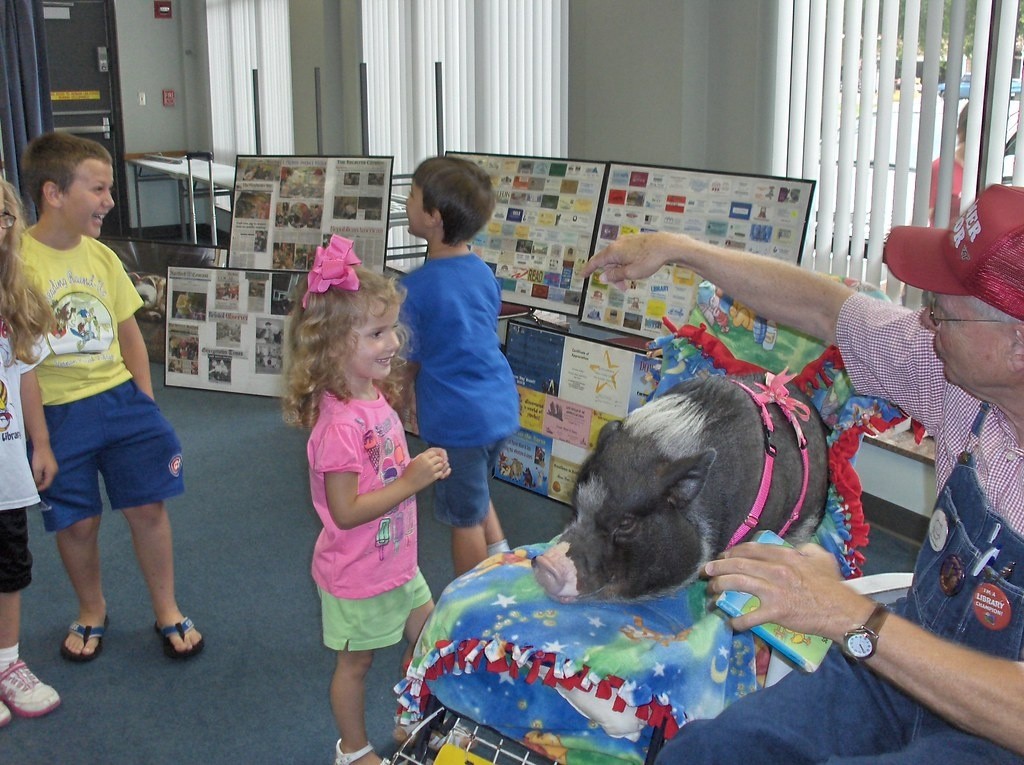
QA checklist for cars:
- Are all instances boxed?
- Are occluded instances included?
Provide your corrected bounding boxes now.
[818,71,1024,190]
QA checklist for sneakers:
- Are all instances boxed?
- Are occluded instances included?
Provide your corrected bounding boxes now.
[0,660,61,728]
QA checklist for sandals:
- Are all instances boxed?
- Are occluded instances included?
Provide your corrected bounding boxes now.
[334,735,390,765]
[393,717,476,754]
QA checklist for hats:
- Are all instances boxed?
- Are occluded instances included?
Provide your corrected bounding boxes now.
[885,185,1024,323]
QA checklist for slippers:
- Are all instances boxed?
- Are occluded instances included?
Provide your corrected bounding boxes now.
[154,619,205,658]
[59,611,110,663]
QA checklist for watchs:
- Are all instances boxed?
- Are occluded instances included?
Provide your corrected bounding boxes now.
[841,602,891,664]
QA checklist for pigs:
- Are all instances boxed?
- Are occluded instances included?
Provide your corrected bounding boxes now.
[533,373,830,604]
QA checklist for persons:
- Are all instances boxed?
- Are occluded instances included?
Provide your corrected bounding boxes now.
[254,230,267,251]
[579,182,1024,765]
[260,321,274,342]
[0,176,62,726]
[19,132,207,663]
[398,155,523,573]
[174,292,193,318]
[282,234,454,764]
[170,334,197,361]
[928,99,969,228]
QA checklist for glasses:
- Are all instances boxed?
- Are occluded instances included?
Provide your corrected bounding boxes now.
[921,288,1024,331]
[0,213,16,229]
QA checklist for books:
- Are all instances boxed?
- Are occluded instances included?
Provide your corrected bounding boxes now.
[714,529,833,673]
